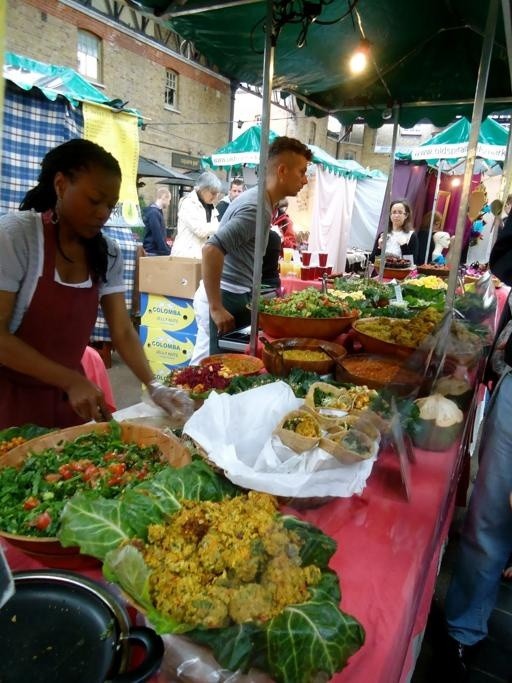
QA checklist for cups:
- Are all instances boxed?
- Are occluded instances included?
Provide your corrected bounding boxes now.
[280,248,333,281]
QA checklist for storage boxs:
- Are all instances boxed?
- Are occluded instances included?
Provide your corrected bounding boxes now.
[137,255,202,380]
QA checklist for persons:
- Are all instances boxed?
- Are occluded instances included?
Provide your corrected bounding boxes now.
[272,197,296,251]
[432,231,451,264]
[0,137,195,429]
[143,173,246,257]
[203,136,314,353]
[417,210,442,263]
[425,210,512,661]
[373,200,418,264]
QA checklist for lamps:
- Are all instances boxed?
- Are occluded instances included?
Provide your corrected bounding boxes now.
[348,40,373,73]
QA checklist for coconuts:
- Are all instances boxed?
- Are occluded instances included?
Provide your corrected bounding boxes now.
[433,375,473,411]
[410,393,464,451]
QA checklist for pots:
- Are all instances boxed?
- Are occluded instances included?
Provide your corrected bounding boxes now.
[1,567,165,682]
